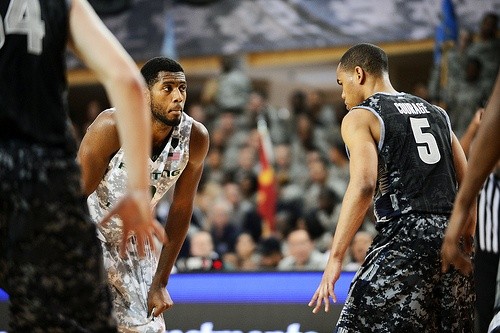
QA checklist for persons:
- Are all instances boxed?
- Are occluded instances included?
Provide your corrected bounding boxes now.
[76,57,210,333]
[442,13,500,333]
[0,0,168,333]
[308,44,477,333]
[77,51,459,271]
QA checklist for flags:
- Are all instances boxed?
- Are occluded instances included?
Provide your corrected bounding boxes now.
[258,120,276,233]
